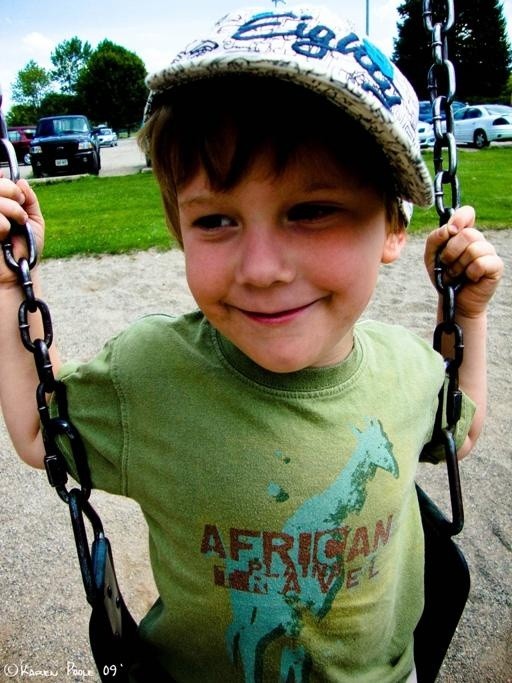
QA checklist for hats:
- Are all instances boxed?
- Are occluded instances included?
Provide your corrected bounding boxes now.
[137,7,436,220]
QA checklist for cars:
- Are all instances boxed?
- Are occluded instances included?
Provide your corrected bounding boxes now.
[1,125,37,166]
[95,127,119,147]
[427,103,512,149]
[417,118,435,151]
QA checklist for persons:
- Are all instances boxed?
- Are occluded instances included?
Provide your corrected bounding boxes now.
[0,6,504,682]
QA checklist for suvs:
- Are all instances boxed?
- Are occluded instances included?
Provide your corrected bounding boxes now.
[418,100,465,128]
[30,114,101,176]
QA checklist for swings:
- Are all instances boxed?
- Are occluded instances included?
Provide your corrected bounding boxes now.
[0,0,471,683]
